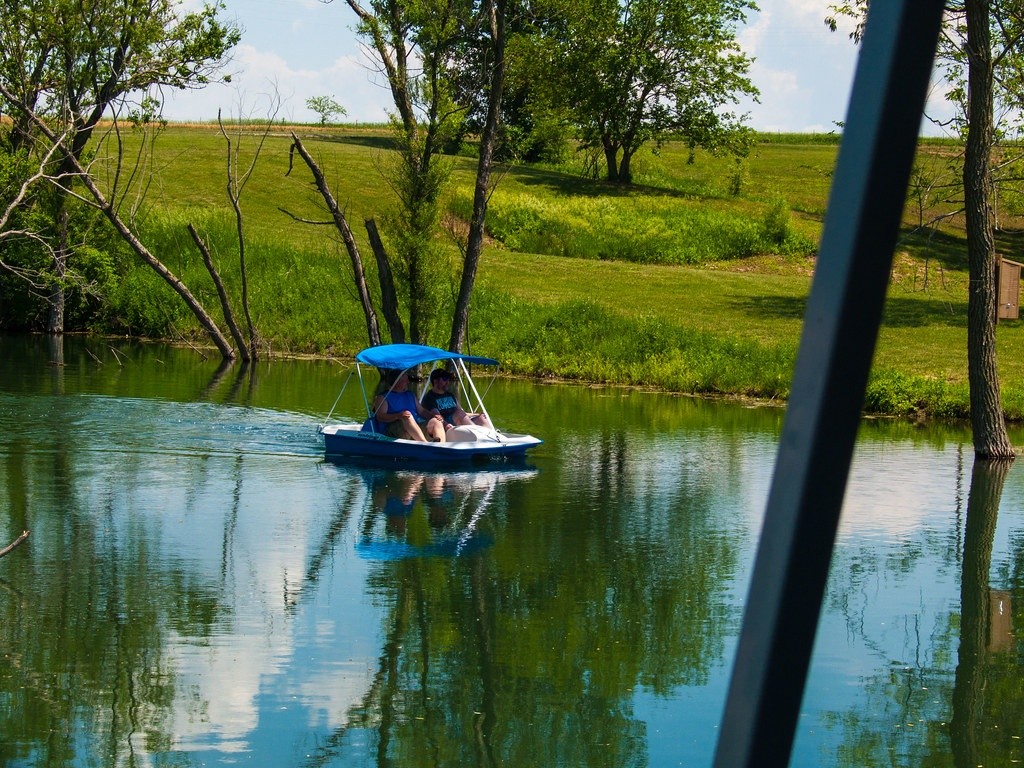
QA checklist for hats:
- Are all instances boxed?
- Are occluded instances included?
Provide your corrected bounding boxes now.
[431,369,451,377]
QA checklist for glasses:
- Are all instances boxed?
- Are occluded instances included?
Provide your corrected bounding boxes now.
[442,377,448,381]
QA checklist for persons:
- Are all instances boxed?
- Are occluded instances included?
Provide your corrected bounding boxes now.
[422,369,492,429]
[374,370,446,442]
[382,474,456,536]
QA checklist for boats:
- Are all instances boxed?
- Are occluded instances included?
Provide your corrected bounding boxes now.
[319,464,539,566]
[319,344,543,472]
